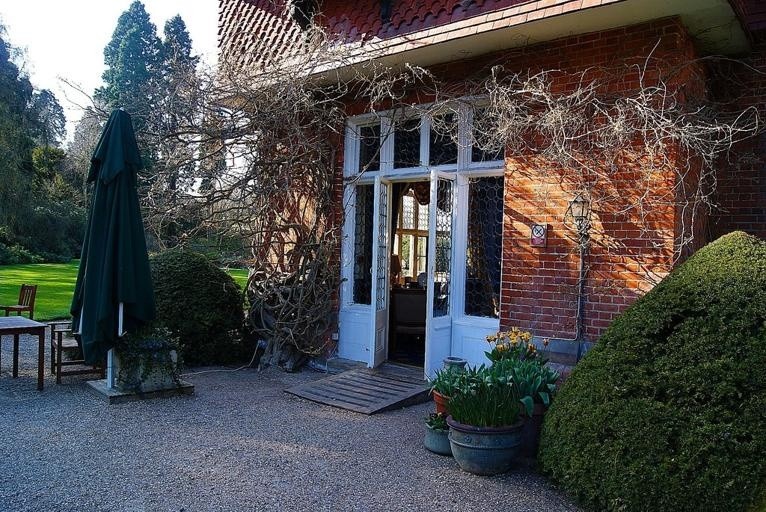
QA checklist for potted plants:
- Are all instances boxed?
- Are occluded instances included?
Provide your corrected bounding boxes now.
[417,356,567,477]
[106,325,184,390]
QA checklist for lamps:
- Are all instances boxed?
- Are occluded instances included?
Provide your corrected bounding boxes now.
[567,189,593,231]
[391,254,402,283]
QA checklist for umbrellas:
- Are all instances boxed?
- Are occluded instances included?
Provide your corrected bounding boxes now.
[71,110,158,391]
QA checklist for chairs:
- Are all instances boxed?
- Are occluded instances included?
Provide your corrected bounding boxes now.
[48,319,107,384]
[1,283,40,319]
[389,280,450,360]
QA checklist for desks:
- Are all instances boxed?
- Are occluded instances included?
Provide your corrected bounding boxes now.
[0,313,49,392]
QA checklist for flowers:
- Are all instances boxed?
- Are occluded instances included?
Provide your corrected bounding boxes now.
[483,325,554,368]
